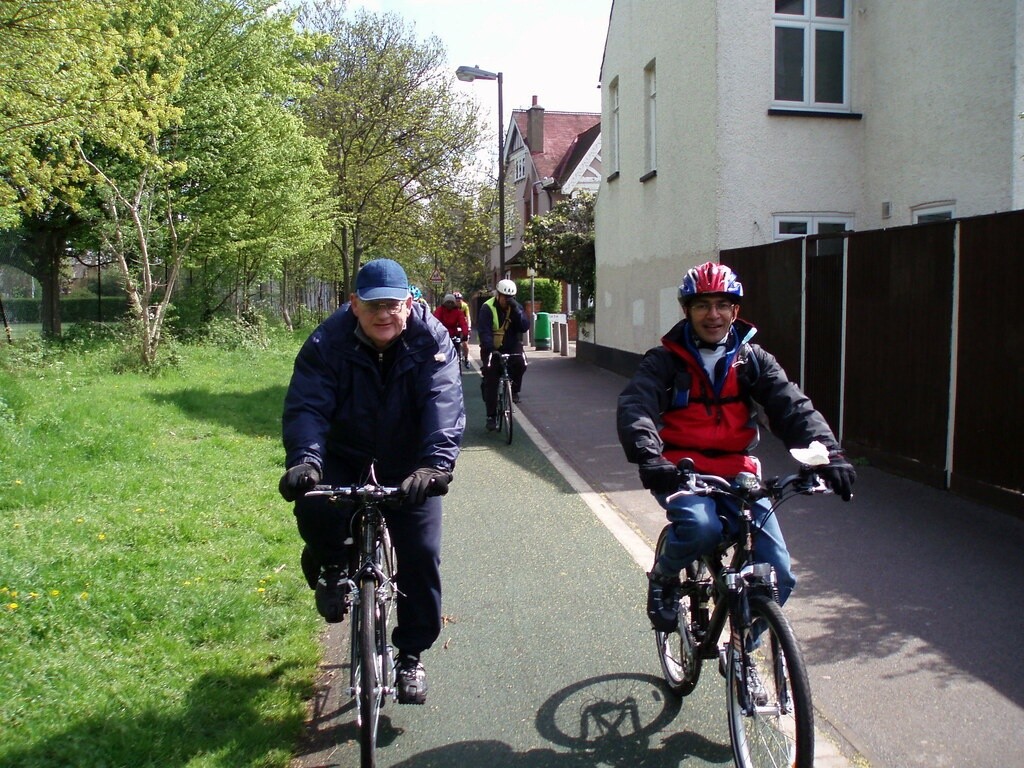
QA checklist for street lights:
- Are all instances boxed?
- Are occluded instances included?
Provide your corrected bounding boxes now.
[456,64,506,281]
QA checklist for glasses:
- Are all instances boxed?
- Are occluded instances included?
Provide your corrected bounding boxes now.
[356,295,407,314]
[686,303,735,315]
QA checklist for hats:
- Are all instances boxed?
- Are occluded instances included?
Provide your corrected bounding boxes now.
[354,259,408,300]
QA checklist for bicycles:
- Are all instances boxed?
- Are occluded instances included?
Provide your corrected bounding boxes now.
[487,346,529,445]
[304,456,442,768]
[650,457,855,768]
[450,330,471,376]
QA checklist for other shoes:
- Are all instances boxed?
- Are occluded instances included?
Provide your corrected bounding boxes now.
[465,359,470,369]
[486,418,496,430]
[512,393,519,403]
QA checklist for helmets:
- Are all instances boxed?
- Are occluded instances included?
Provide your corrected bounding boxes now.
[409,285,420,300]
[677,262,743,308]
[442,294,457,304]
[453,292,463,299]
[496,279,517,295]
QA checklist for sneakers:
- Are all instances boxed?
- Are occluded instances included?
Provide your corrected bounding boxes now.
[720,647,767,708]
[315,566,346,618]
[646,563,680,632]
[392,654,427,703]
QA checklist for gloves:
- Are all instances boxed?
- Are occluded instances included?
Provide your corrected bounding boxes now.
[799,447,855,503]
[507,296,521,313]
[400,464,453,504]
[279,463,320,502]
[491,350,501,361]
[637,446,691,495]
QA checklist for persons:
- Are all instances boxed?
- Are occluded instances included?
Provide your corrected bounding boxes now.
[279,259,466,702]
[434,292,471,368]
[408,286,430,311]
[617,262,859,710]
[478,280,530,430]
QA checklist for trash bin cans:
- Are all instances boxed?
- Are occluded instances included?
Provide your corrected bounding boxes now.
[534,312,551,350]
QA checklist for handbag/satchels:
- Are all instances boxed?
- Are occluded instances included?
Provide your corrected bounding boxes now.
[492,329,505,349]
